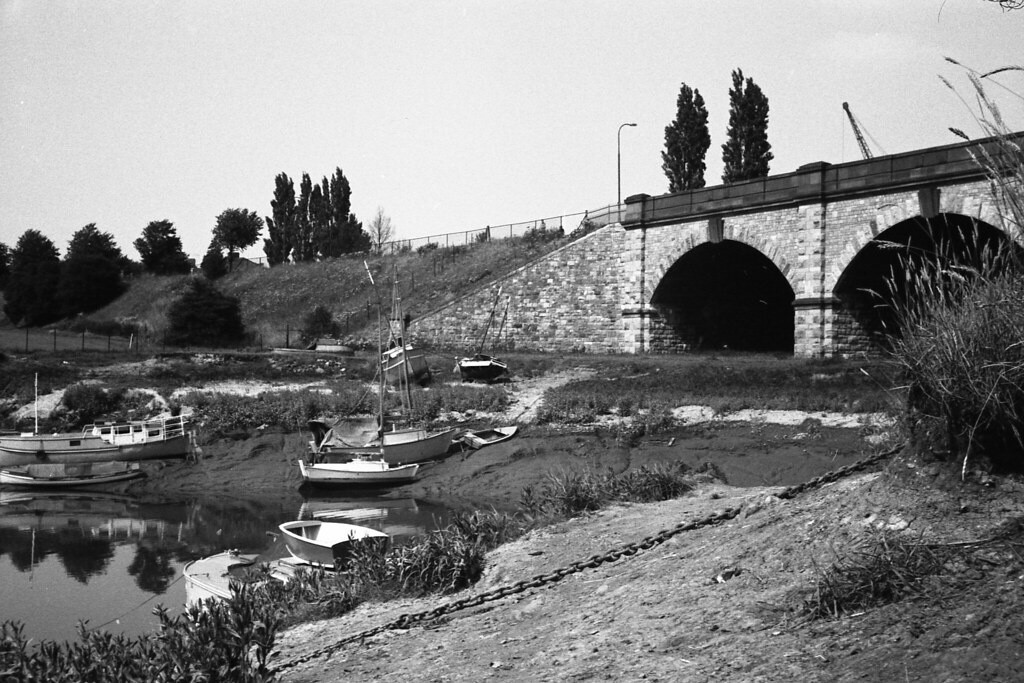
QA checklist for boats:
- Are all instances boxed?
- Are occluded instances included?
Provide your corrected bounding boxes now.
[1,490,139,512]
[309,264,458,466]
[296,286,420,483]
[279,516,390,570]
[454,280,509,381]
[0,469,148,490]
[183,548,306,621]
[462,424,521,450]
[361,257,429,384]
[1,371,199,465]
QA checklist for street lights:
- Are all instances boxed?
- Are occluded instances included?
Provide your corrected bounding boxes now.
[616,122,639,226]
